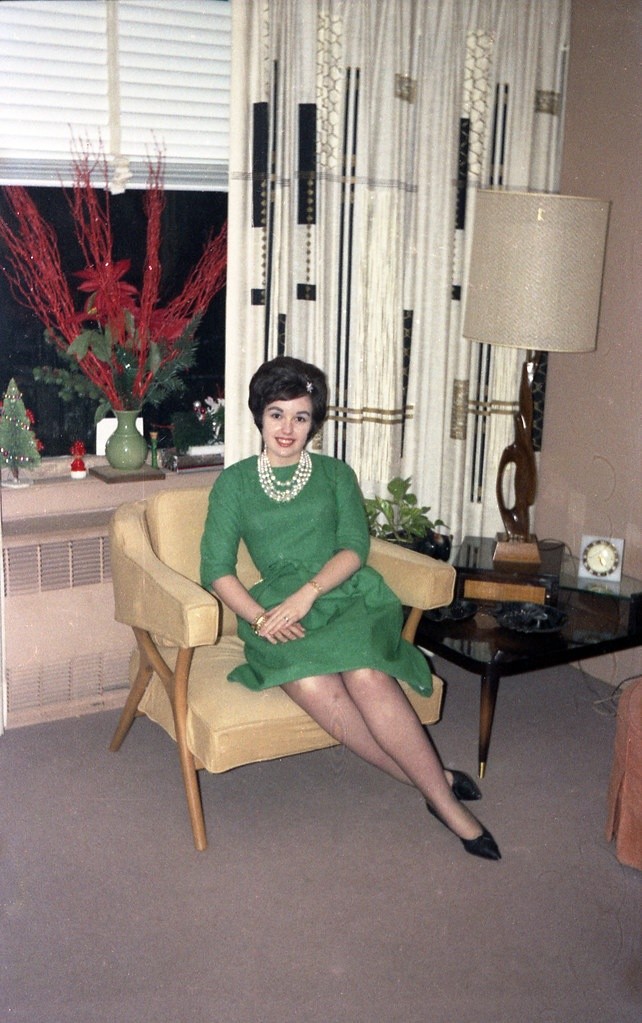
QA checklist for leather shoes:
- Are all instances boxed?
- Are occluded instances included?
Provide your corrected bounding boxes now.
[426,796,501,860]
[442,768,482,801]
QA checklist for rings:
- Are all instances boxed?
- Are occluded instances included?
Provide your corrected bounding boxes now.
[284,617,289,622]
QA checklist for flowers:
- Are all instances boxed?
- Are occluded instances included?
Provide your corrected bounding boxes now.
[0,121,227,430]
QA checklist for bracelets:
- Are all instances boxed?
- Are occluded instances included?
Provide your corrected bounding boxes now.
[307,580,324,595]
[252,610,270,636]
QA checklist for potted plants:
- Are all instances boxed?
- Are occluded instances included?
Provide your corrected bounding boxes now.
[364,476,454,562]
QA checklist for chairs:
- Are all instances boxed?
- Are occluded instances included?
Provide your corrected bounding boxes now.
[109,484,456,851]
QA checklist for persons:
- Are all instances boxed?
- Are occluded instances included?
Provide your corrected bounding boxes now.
[200,356,502,861]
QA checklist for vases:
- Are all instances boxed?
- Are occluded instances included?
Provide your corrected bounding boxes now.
[105,409,148,471]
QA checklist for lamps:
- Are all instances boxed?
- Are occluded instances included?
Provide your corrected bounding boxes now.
[453,187,612,608]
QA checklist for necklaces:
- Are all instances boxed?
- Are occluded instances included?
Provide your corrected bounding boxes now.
[257,442,312,503]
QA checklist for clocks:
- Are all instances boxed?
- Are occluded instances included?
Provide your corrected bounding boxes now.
[578,535,624,583]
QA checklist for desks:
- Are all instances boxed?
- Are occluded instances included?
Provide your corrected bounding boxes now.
[379,530,642,780]
